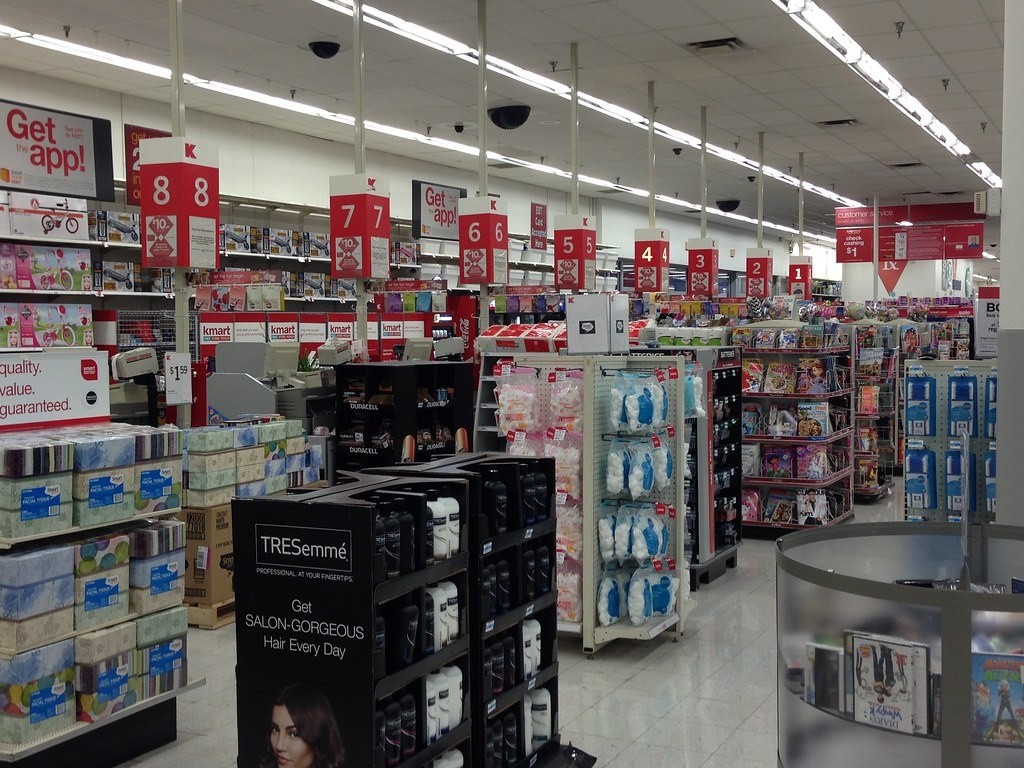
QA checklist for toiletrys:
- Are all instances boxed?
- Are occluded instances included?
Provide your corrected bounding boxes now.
[374,581,460,680]
[480,460,547,537]
[367,487,460,584]
[480,542,551,621]
[482,619,542,703]
[485,687,552,767]
[374,666,463,768]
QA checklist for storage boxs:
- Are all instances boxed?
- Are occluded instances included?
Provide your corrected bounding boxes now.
[1,189,619,744]
[0,302,21,348]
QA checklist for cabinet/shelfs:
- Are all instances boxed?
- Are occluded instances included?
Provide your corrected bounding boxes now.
[0,241,207,767]
[474,351,558,451]
[218,449,598,768]
[728,326,855,532]
[89,177,840,348]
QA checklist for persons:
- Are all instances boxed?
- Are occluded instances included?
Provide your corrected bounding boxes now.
[806,360,828,394]
[261,683,346,768]
[802,489,826,525]
